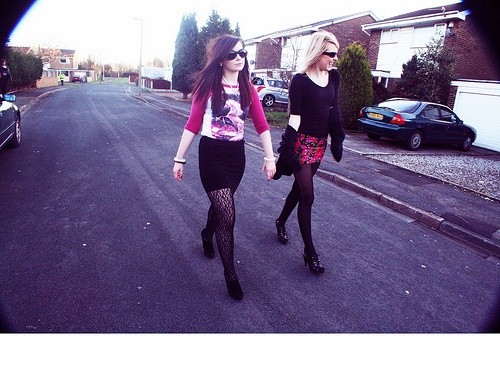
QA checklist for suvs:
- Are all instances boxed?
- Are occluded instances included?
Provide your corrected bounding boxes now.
[251,75,291,108]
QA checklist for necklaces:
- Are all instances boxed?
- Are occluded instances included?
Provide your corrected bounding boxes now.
[222,75,240,93]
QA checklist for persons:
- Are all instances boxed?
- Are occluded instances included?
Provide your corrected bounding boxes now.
[172,36,277,301]
[59,72,65,85]
[0,58,12,95]
[272,30,345,272]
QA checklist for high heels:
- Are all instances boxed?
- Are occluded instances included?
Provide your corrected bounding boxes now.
[275,218,288,243]
[302,249,324,272]
[223,268,243,300]
[201,229,214,259]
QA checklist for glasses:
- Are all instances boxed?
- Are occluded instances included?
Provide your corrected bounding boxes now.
[322,51,338,58]
[226,49,247,61]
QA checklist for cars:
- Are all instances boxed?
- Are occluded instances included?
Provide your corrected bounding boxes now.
[357,97,477,152]
[0,92,22,151]
[71,75,82,83]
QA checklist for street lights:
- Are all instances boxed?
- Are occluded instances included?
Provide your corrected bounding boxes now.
[134,17,143,96]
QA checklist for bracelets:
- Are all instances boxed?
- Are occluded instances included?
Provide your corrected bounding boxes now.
[264,157,275,161]
[173,157,186,164]
